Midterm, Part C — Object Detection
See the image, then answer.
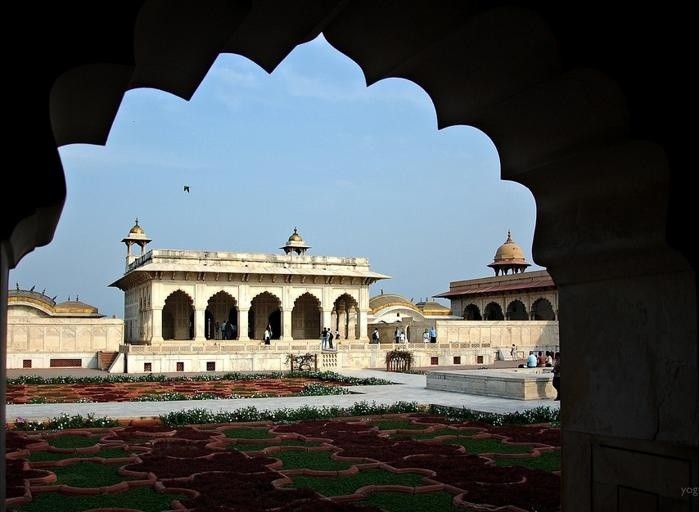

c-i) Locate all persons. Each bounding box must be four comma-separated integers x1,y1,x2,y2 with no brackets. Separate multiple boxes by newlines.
327,328,333,349
537,351,546,367
215,320,237,340
527,351,537,368
335,329,340,339
545,351,553,367
400,332,405,342
372,328,380,343
429,326,436,343
321,327,328,350
552,352,560,401
422,327,429,343
510,344,517,362
395,326,401,343
264,323,273,345
